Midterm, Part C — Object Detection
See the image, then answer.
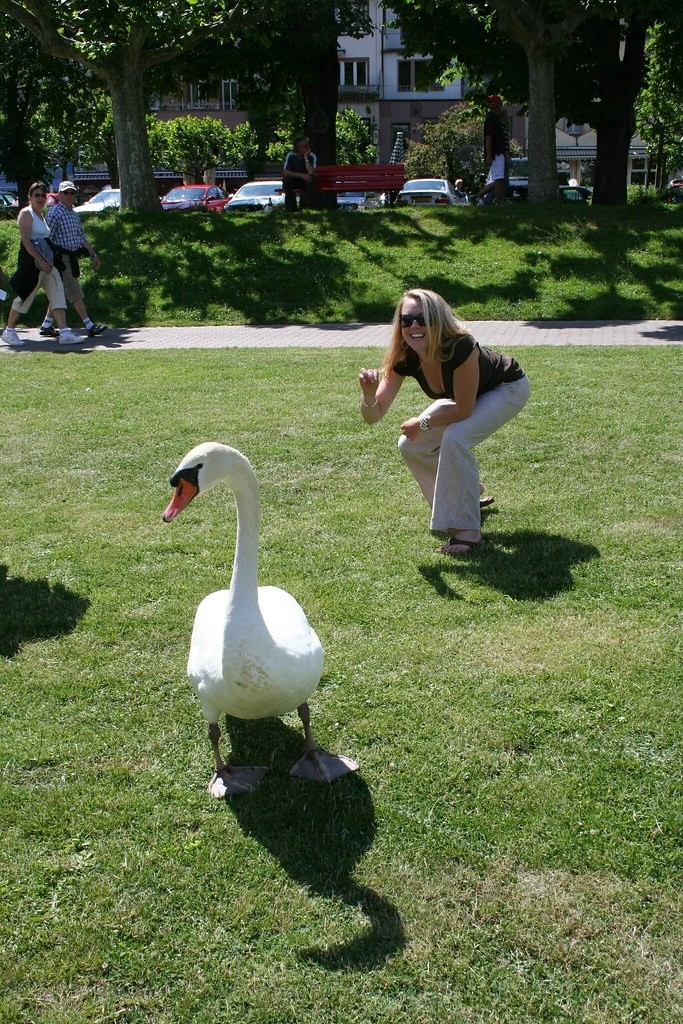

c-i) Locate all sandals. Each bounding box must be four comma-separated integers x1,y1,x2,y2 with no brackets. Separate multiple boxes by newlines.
39,325,60,336
86,324,107,337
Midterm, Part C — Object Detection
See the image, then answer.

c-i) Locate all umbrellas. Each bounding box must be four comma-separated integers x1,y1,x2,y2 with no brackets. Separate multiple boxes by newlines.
389,132,404,164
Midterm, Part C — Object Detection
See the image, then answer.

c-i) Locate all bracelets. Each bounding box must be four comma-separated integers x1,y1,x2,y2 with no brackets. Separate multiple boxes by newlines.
305,160,309,162
91,253,97,260
419,417,432,432
361,394,378,408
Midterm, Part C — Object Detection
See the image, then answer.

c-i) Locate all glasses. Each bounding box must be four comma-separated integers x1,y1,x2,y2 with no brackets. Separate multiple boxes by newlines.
399,312,436,329
64,191,76,196
32,193,46,198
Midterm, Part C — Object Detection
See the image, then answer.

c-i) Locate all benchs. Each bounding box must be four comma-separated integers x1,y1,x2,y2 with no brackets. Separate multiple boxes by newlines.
274,164,405,209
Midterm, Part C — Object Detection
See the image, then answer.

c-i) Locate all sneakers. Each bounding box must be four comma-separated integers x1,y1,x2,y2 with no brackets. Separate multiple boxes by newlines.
468,194,480,206
2,330,26,346
59,333,85,345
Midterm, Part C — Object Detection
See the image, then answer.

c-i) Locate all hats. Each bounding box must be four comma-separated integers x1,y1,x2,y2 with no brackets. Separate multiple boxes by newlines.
489,96,502,105
58,181,78,192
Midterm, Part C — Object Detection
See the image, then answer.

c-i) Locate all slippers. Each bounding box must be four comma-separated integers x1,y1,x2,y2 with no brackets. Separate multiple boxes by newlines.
478,495,494,511
435,536,483,556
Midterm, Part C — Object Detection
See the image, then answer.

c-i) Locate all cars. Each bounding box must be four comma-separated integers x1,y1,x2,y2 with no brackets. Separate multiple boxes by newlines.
161,185,232,213
379,190,398,209
73,189,121,212
11,194,60,207
481,180,593,204
663,179,683,203
337,190,382,210
395,179,466,205
0,191,20,211
223,180,300,212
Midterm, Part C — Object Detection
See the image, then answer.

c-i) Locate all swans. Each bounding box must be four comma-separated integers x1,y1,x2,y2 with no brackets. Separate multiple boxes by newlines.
162,441,362,798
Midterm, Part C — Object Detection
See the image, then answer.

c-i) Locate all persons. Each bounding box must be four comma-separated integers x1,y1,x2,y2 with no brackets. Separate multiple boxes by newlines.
39,181,108,338
470,95,512,206
359,289,530,553
2,181,84,345
568,174,579,187
283,136,316,213
454,179,468,203
